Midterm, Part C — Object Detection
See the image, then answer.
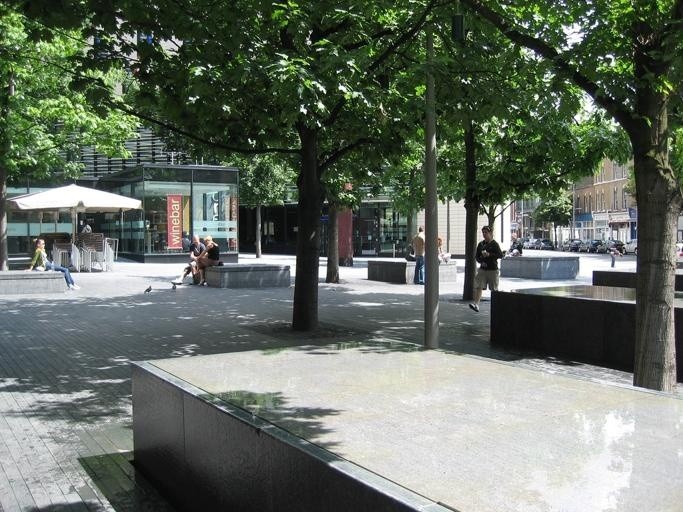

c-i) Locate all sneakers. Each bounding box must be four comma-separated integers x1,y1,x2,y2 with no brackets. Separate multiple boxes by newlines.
171,278,183,285
469,303,480,312
67,283,80,290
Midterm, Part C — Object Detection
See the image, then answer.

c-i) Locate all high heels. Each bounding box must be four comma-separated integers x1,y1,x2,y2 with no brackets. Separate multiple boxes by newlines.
196,280,206,286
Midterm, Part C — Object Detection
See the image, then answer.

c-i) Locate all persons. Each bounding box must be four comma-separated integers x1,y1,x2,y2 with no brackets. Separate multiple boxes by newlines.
172,235,205,285
609,242,623,267
196,235,219,286
80,220,92,233
509,233,522,256
25,239,80,292
469,226,503,312
412,227,425,285
437,238,449,264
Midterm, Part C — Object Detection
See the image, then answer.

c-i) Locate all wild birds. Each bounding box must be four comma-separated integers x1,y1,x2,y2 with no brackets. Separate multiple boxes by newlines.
143,285,152,295
172,283,176,290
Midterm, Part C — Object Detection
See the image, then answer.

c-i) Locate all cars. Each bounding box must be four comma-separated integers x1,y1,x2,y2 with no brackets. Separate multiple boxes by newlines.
675,242,682,263
520,237,637,256
